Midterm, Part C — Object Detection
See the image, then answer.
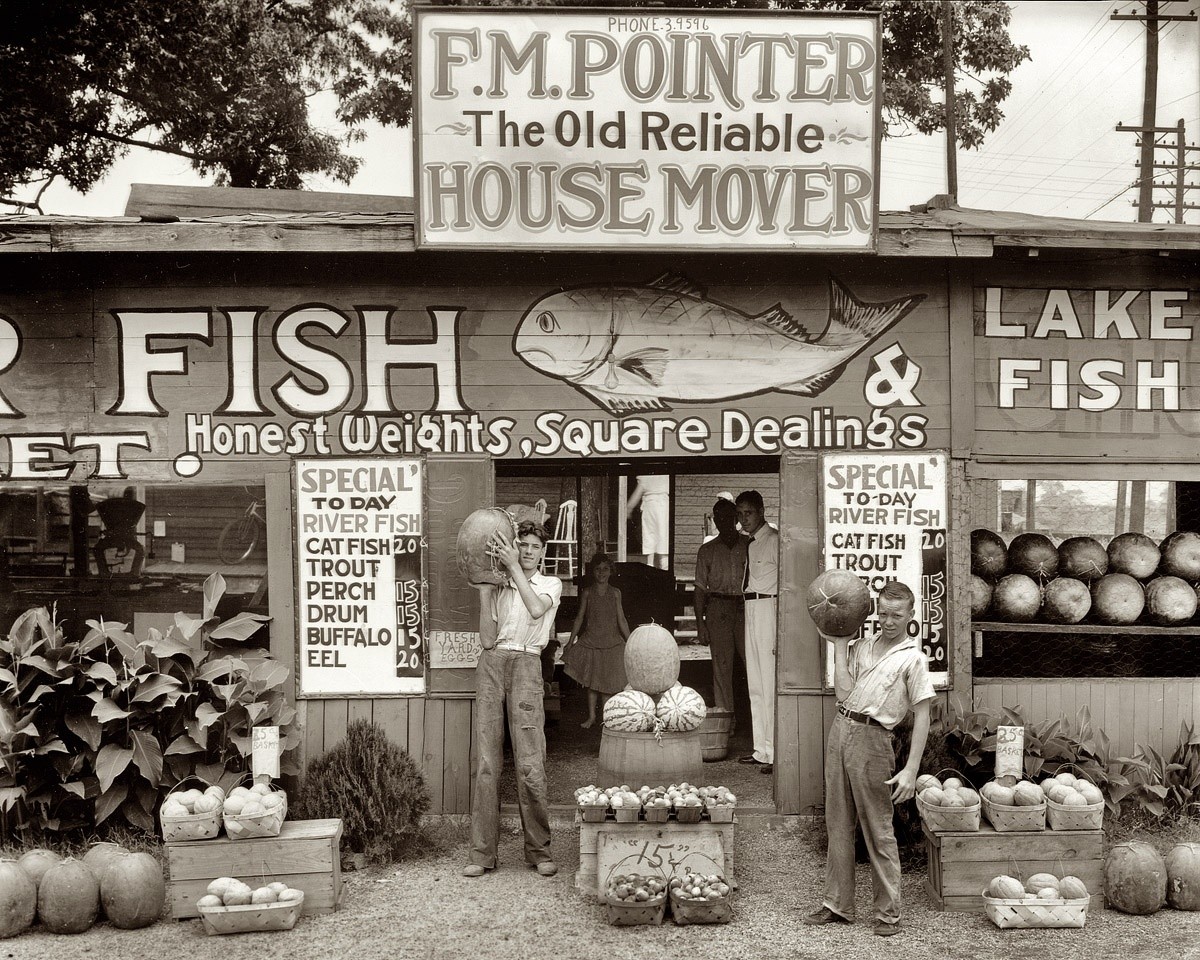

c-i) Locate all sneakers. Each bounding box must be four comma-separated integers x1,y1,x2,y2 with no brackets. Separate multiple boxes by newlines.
804,906,843,925
875,919,901,936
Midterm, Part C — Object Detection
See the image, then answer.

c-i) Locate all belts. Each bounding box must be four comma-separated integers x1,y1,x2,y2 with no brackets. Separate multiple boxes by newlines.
838,706,881,726
711,592,742,601
495,642,542,656
745,593,777,600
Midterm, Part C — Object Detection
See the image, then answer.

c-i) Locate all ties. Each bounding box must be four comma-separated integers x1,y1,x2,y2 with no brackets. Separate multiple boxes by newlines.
743,536,755,593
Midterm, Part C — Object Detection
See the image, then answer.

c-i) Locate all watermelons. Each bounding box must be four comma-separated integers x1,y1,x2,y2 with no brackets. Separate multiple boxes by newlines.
807,569,871,636
969,528,1200,626
603,617,707,732
456,507,517,584
1104,840,1200,914
0,842,166,939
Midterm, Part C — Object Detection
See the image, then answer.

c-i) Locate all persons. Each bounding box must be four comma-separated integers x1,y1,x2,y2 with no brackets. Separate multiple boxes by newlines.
626,475,670,570
93,486,146,579
735,490,781,774
558,551,633,729
692,498,753,737
803,582,938,934
462,521,562,878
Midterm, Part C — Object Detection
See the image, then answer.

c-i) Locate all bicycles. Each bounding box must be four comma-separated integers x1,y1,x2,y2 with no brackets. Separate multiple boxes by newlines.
218,484,266,565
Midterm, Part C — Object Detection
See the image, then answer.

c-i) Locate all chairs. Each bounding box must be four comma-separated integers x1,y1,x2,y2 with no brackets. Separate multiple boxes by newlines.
532,497,580,578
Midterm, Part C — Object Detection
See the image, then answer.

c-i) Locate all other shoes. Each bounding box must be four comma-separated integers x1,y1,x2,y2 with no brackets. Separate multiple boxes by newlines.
536,861,558,876
738,756,764,764
761,765,773,774
464,865,484,877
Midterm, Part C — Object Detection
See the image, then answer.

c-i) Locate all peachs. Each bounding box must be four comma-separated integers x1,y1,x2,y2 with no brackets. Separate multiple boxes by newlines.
608,874,666,903
670,872,728,900
574,784,737,807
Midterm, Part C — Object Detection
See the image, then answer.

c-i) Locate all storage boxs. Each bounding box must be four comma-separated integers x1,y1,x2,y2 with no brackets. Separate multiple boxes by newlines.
162,817,349,921
922,820,1113,918
573,806,740,896
